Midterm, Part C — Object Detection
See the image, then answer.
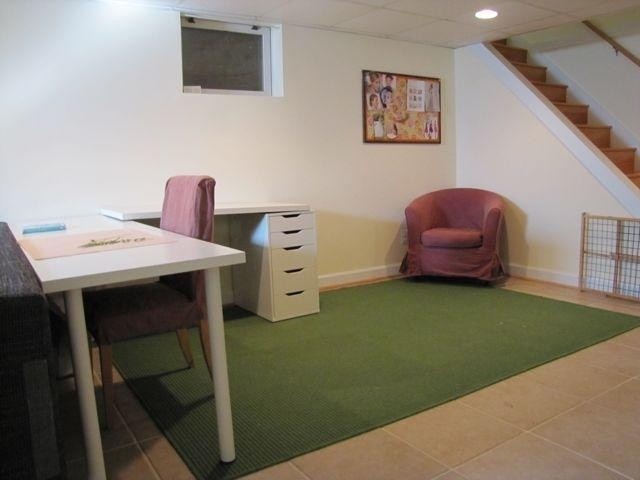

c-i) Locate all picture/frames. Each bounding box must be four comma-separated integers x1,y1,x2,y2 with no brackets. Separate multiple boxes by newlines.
362,68,442,145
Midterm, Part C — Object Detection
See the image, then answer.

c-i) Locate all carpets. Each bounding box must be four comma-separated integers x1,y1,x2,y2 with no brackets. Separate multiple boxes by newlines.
108,277,639,480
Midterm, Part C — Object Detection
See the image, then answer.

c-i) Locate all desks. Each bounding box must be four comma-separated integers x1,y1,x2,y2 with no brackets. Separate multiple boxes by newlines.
98,201,319,322
6,215,246,480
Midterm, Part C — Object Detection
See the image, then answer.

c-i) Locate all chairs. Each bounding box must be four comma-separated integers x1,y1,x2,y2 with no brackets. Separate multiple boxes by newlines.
398,188,506,289
64,176,216,432
